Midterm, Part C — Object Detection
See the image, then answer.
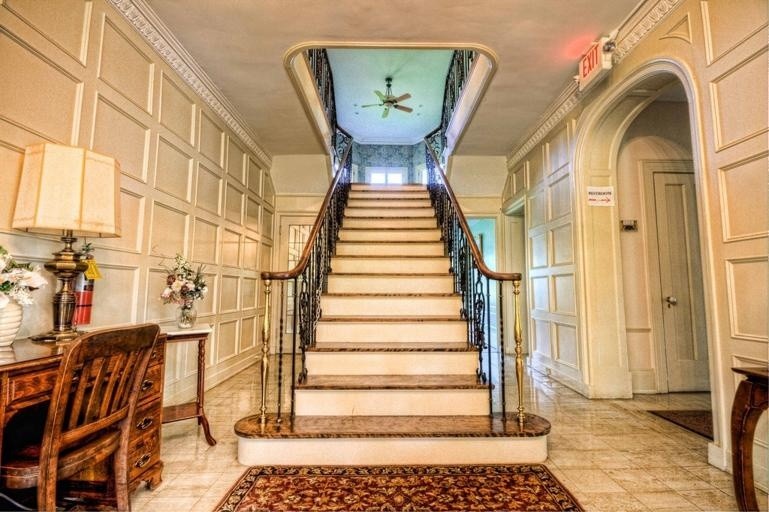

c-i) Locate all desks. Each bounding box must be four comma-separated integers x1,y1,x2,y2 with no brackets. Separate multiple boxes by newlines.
151,321,218,446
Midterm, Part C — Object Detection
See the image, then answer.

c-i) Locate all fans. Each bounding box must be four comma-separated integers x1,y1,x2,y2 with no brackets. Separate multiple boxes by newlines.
361,77,414,119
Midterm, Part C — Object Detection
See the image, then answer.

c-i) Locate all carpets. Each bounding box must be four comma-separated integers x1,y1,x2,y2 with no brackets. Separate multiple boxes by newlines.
646,409,713,440
210,465,588,512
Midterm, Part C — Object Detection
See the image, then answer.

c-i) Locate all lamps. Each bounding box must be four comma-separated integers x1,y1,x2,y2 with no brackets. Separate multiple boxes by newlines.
12,142,123,344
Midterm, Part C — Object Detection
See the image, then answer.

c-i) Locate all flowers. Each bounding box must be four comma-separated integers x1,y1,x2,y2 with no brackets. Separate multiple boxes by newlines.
0,246,49,309
157,251,209,325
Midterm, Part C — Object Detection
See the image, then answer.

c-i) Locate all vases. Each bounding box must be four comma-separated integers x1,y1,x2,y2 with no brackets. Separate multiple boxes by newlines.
175,304,197,329
0,298,23,346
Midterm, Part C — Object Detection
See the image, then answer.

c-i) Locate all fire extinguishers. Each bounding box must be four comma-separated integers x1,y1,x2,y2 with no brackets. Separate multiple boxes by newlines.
72,241,95,327
